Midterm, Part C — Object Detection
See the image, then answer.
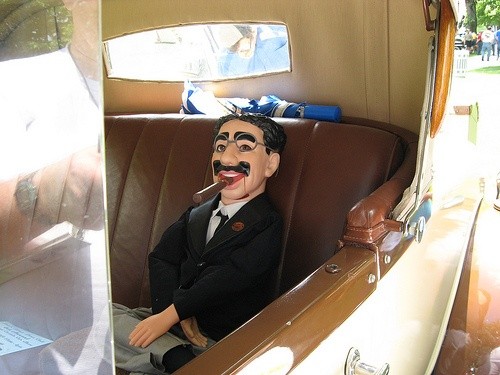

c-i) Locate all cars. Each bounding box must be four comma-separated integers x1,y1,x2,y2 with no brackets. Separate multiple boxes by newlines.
1,1,487,375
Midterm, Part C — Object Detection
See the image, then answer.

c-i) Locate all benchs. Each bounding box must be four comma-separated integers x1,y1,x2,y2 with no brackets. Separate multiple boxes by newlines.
0,114,419,375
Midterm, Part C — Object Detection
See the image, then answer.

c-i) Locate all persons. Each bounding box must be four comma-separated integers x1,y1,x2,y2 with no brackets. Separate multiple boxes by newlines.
455,27,500,61
128,113,286,354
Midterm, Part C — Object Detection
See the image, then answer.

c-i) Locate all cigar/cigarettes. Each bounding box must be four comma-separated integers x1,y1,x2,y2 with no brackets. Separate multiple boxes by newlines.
191,177,234,203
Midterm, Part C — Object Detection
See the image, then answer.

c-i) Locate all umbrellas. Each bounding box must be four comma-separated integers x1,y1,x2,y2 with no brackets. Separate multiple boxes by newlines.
179,81,343,124
